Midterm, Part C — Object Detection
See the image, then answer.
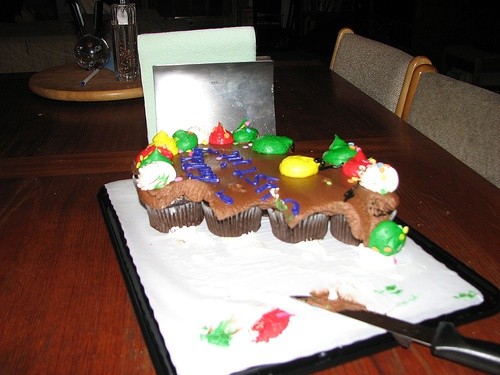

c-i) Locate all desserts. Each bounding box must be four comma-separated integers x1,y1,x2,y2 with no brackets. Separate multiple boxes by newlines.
130,118,409,256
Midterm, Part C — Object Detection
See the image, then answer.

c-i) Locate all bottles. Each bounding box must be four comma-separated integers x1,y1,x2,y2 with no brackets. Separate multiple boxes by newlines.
110,0,140,81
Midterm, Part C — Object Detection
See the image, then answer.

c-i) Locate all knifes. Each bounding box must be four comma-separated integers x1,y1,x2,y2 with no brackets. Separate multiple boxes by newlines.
291,296,500,375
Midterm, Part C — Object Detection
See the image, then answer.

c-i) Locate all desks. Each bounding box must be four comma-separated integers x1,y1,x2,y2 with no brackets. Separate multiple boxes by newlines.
0,58,500,374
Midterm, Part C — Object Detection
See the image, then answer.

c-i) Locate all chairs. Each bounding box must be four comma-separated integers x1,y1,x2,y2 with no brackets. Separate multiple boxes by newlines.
327,27,500,190
